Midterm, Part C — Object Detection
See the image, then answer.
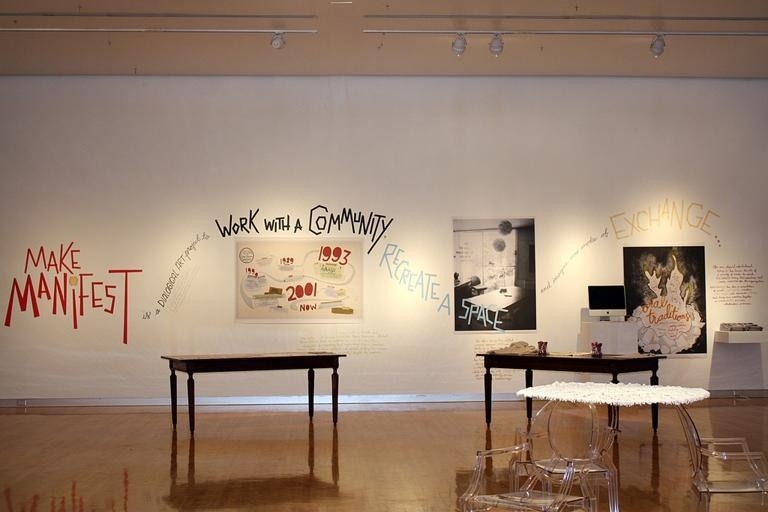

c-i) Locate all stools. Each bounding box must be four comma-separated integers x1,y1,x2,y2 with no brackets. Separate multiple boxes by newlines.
470,286,487,296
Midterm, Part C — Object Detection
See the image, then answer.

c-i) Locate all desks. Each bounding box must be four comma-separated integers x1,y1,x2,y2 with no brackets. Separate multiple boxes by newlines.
464,285,531,322
475,350,667,432
162,352,347,433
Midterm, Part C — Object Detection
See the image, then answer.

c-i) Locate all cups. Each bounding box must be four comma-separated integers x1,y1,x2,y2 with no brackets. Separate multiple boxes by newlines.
537,341,548,354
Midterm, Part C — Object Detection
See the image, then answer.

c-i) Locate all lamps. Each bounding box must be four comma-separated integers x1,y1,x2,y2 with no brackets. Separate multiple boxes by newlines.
488,33,504,55
452,35,466,53
271,33,284,49
650,37,667,56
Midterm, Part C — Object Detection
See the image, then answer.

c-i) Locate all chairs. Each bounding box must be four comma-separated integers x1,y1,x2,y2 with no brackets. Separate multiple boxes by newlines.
457,400,600,512
675,403,768,512
516,403,620,512
